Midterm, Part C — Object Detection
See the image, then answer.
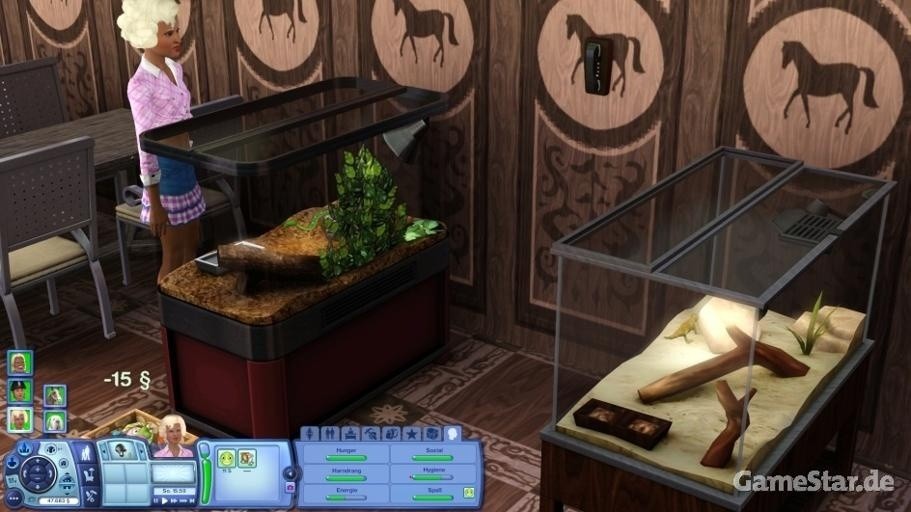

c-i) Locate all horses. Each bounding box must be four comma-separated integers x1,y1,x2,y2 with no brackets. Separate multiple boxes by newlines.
259,0,307,42
566,16,645,97
782,42,879,134
393,0,459,67
